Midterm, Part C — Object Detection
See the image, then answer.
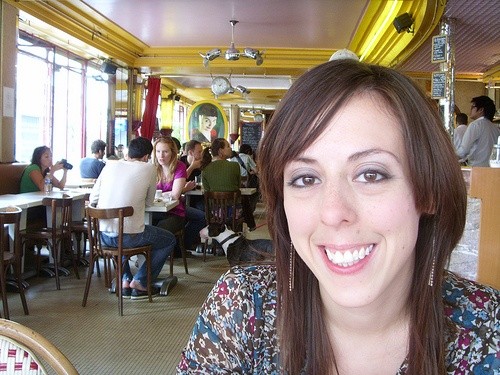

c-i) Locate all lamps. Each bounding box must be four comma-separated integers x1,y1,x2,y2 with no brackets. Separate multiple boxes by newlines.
200,19,264,66
228,86,246,93
99,58,119,74
393,14,413,33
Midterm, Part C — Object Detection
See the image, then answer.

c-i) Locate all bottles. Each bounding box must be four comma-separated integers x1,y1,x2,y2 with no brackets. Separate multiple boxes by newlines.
43,172,53,196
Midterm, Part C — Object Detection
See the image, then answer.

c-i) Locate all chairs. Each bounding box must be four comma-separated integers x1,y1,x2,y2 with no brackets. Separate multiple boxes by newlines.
1,318,80,375
202,191,244,260
0,193,152,321
170,193,189,277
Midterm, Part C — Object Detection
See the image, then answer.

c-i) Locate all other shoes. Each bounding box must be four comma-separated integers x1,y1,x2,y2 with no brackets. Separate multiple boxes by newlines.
121,288,131,298
173,251,191,257
131,288,157,299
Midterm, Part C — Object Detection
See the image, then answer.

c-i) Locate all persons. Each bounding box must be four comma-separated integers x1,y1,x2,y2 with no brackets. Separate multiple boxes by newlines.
79,139,106,179
89,137,174,300
175,59,500,375
20,145,67,229
151,138,188,234
191,104,218,143
454,95,500,167
171,137,259,253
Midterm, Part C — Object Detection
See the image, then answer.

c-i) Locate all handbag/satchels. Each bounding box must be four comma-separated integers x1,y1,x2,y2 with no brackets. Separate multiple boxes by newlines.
246,174,259,199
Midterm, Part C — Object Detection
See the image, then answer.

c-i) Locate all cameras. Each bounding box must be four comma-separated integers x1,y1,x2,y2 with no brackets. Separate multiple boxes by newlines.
59,159,72,169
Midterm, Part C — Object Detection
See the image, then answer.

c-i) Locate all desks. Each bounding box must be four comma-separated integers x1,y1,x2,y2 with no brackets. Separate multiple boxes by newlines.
109,198,180,296
183,188,257,253
0,182,95,292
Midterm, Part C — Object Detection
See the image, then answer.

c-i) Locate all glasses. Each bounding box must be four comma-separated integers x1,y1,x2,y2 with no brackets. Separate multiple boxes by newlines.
471,106,476,110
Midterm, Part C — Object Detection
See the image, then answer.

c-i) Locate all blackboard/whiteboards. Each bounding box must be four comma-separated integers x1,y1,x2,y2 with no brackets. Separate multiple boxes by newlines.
431,34,448,63
239,121,263,152
431,71,446,99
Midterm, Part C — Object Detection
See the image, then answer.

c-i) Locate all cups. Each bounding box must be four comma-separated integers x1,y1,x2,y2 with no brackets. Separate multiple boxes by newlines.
155,190,162,199
195,176,201,189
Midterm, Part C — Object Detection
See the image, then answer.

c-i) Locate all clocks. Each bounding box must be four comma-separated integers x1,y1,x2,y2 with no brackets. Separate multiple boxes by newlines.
211,76,231,96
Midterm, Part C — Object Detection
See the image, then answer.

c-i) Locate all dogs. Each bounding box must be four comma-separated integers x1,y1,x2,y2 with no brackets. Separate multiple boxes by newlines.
199,222,277,266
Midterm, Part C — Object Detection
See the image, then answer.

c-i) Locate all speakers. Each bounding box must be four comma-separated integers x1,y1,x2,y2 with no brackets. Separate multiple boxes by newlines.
102,62,117,74
393,12,413,34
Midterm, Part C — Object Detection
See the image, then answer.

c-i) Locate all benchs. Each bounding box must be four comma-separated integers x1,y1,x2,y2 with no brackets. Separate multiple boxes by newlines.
0,162,46,251
